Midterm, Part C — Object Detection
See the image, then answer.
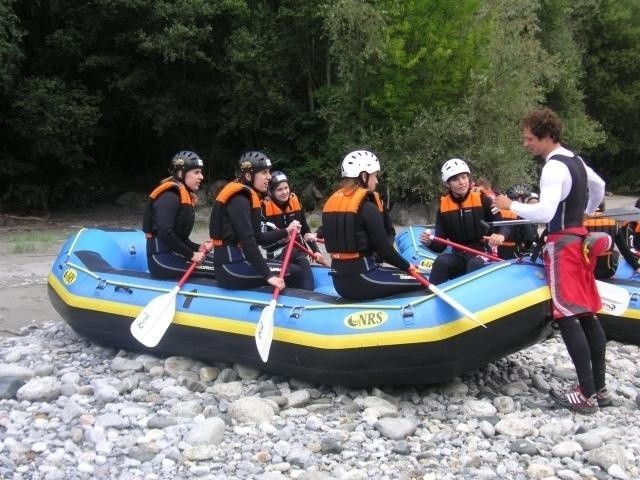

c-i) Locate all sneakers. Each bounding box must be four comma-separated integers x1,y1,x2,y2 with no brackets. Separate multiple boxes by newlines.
549,384,612,414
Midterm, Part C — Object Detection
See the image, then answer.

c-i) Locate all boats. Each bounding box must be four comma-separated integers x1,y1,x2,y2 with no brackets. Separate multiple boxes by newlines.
47,226,554,389
394,215,640,348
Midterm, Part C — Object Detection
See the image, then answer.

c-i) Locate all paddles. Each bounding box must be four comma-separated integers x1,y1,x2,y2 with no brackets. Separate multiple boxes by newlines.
431,234,630,316
254,228,297,363
129,245,207,349
411,267,487,329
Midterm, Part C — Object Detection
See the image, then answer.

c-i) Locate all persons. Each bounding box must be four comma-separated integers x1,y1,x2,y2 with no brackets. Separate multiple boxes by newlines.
470,178,640,279
420,158,510,286
210,150,314,291
142,150,215,280
304,190,396,263
258,171,328,290
494,108,612,413
322,150,428,301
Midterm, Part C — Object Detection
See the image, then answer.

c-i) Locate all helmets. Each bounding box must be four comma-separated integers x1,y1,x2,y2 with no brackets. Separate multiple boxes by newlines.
506,184,530,200
236,151,288,189
341,150,380,178
169,151,204,181
440,159,470,182
